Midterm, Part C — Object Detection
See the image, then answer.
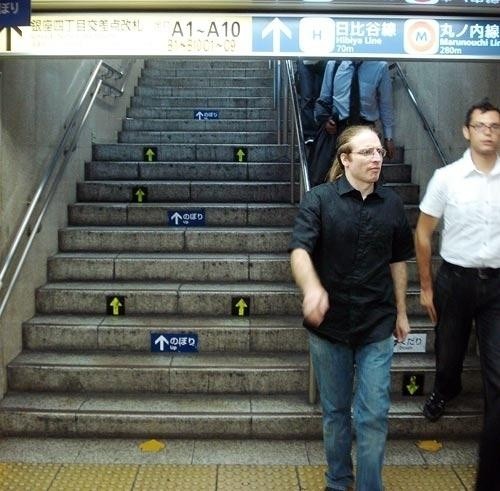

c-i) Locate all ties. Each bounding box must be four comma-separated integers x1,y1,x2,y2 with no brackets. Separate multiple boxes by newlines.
349,60,364,125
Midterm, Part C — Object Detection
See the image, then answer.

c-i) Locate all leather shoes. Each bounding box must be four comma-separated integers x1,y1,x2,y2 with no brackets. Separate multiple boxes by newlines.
423,388,448,423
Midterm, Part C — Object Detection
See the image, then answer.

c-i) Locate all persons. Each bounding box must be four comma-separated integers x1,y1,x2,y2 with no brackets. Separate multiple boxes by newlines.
287,126,417,491
314,59,395,182
413,100,500,491
298,58,326,144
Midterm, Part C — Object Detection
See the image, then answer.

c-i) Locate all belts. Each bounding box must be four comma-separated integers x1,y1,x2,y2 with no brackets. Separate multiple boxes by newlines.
446,266,500,280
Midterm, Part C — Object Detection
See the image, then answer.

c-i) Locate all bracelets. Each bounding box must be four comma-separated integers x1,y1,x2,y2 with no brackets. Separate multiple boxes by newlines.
384,137,394,142
